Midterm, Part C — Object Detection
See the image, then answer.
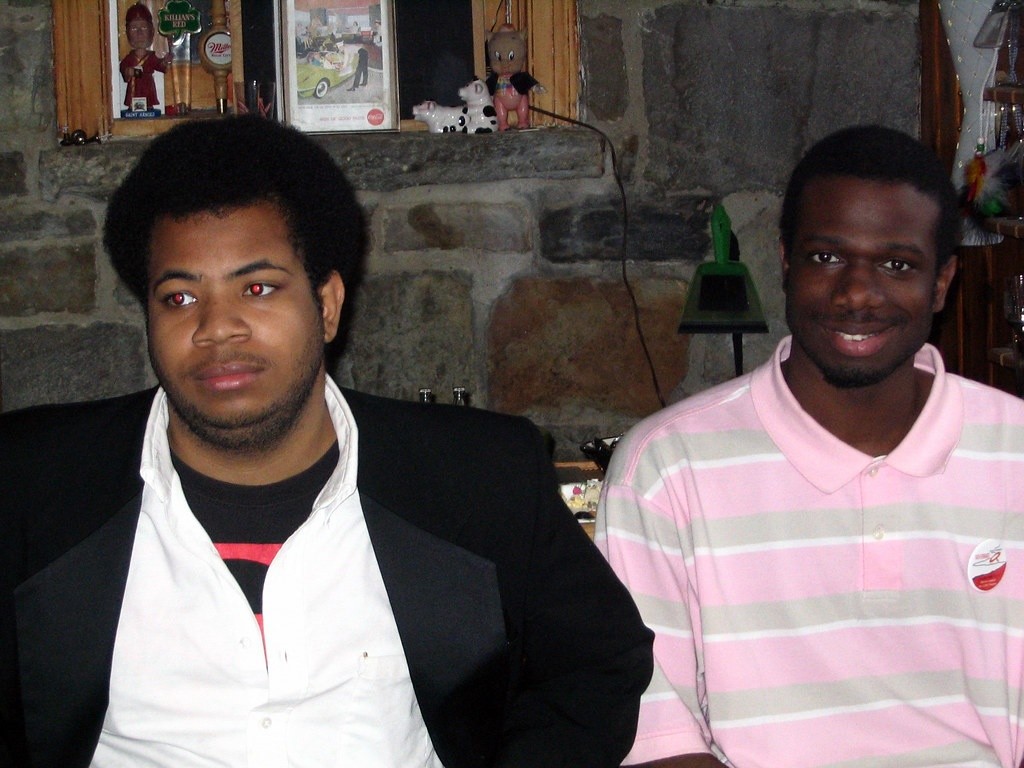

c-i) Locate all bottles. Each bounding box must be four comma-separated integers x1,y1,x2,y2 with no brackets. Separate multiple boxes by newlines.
451,386,466,406
419,388,431,404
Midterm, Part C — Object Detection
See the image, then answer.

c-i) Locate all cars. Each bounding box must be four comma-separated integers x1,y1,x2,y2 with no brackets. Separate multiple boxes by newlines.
296,44,360,99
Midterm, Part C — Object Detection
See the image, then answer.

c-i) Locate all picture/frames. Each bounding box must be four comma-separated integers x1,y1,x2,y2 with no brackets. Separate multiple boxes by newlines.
280,0,402,135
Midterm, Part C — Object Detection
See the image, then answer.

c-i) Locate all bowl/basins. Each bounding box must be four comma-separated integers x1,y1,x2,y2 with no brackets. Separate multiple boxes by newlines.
580,436,623,475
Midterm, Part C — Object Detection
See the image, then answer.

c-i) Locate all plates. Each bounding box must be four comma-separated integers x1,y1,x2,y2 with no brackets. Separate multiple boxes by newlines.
558,481,596,523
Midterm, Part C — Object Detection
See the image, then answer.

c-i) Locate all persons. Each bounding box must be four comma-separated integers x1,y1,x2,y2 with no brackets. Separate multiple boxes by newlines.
594,124,1024,768
120,3,173,111
0,113,654,768
